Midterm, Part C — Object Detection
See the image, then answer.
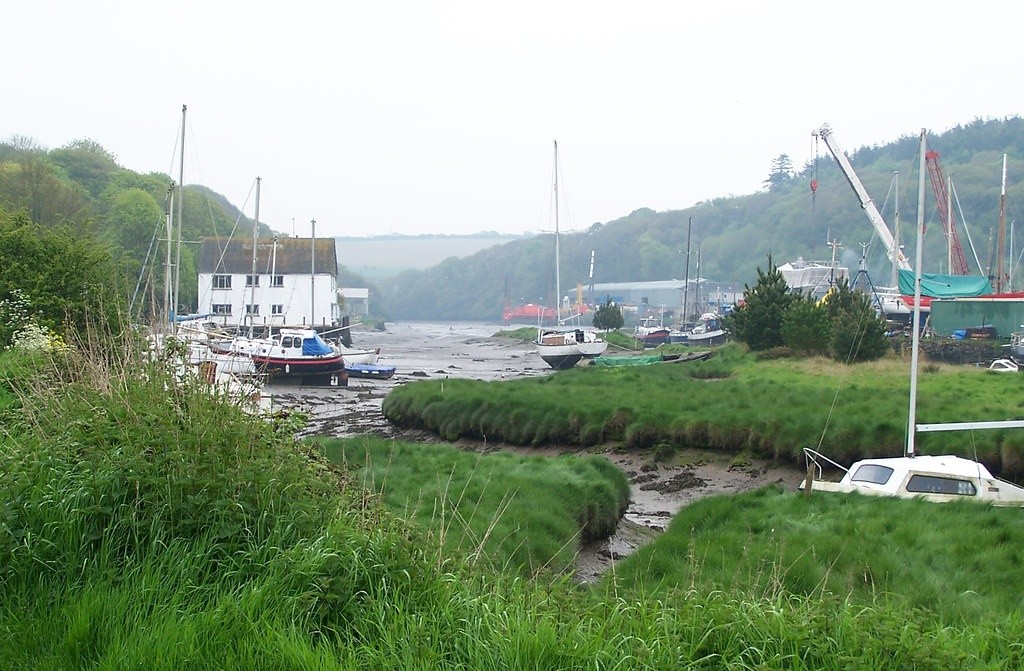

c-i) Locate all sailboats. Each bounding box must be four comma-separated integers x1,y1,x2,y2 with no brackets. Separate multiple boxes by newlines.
532,141,608,371
797,128,1024,511
119,104,397,413
667,217,735,348
853,169,912,329
897,172,995,331
930,154,1024,340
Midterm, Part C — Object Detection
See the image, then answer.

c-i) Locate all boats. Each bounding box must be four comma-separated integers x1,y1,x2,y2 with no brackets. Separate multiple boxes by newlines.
219,328,349,387
773,227,851,304
633,306,670,349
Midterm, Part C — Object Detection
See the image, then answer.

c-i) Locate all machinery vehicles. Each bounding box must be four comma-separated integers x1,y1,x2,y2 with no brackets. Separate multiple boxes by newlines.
812,123,913,272
